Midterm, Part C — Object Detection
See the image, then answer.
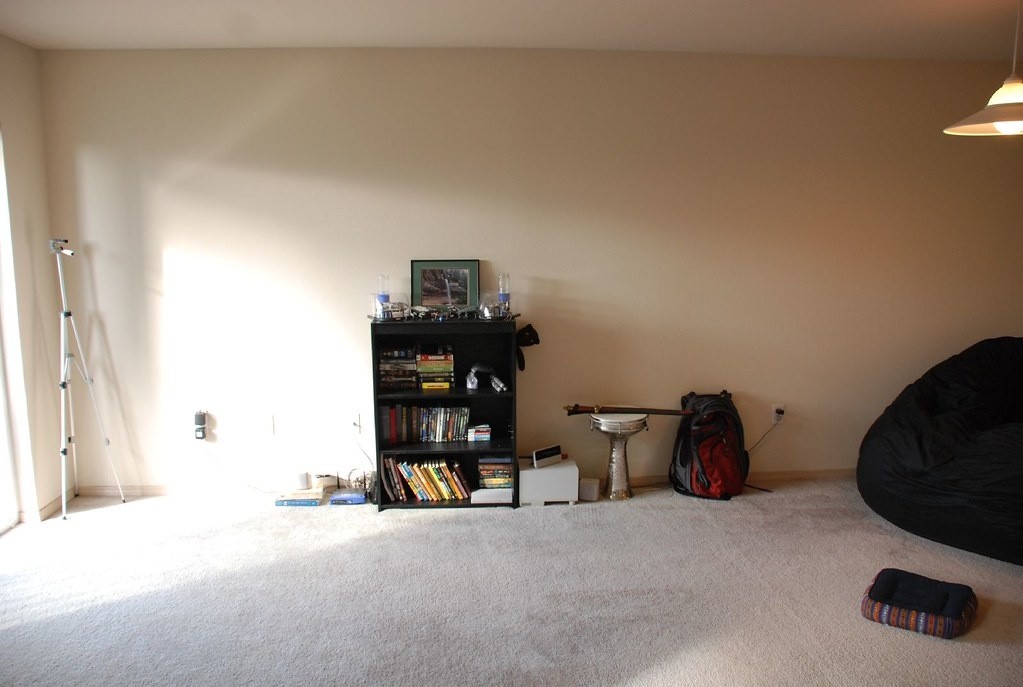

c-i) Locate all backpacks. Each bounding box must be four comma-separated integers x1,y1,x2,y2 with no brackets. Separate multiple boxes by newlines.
670,391,750,501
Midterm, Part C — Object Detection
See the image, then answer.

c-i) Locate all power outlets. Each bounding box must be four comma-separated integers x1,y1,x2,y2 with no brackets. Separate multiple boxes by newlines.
771,403,785,426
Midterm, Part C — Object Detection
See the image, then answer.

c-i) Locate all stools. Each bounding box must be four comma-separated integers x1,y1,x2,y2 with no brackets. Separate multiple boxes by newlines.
518,458,579,508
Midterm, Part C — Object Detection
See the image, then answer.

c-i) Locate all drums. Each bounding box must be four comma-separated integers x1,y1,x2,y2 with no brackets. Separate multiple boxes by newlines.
589,413,649,500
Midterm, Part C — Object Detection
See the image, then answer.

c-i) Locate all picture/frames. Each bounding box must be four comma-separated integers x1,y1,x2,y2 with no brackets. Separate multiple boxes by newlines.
410,259,479,312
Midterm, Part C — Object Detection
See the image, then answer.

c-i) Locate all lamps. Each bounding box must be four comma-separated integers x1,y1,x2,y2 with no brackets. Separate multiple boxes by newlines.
943,0,1023,136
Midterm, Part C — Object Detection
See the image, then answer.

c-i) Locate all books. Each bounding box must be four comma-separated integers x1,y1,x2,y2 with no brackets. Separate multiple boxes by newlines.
378,340,455,390
377,401,491,444
275,489,325,506
379,452,514,503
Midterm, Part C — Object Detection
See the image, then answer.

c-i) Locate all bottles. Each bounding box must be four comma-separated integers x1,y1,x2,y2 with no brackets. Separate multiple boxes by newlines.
498,272,510,307
377,273,389,304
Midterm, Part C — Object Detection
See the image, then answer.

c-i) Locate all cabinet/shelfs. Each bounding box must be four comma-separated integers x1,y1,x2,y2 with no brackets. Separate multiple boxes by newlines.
370,318,520,513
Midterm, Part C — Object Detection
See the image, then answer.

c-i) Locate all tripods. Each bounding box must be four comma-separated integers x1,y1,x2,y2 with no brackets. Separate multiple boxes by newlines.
56,252,126,519
